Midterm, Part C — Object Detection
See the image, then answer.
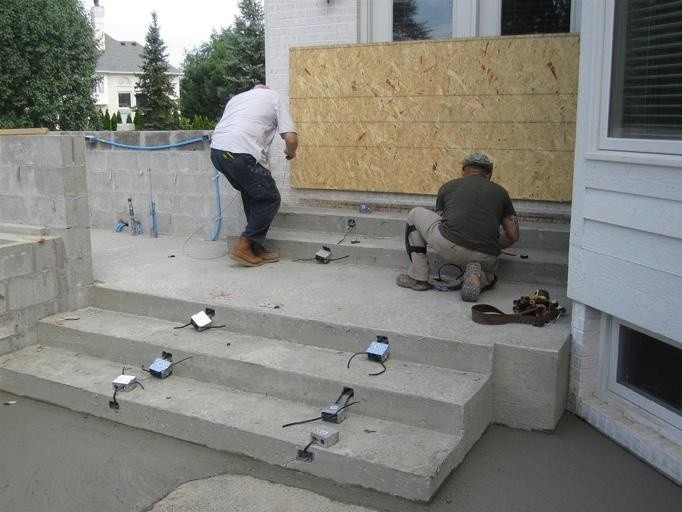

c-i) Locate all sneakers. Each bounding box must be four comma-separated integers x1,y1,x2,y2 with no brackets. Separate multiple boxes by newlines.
396,274,430,291
461,261,482,302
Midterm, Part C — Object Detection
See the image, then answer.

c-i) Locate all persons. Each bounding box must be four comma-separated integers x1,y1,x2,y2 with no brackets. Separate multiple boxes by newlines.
394,151,521,302
209,82,299,267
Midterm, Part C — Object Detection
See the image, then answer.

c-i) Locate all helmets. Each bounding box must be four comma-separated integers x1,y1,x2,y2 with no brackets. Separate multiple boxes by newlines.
462,152,493,179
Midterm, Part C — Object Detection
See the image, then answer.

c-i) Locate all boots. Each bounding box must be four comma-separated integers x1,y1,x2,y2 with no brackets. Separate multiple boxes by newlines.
228,235,279,267
